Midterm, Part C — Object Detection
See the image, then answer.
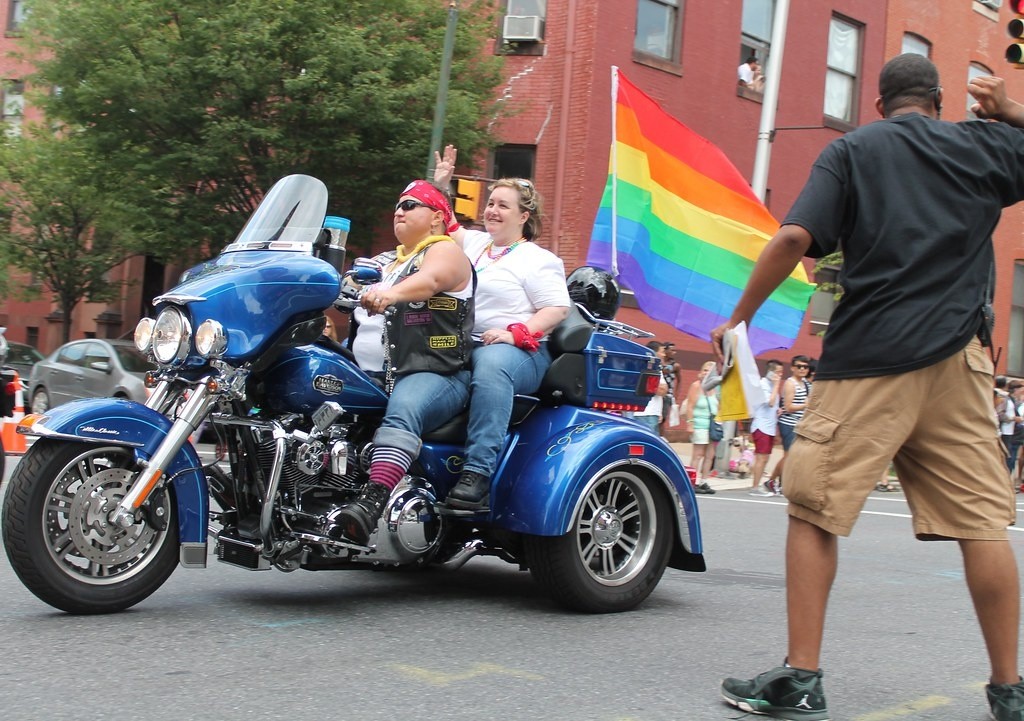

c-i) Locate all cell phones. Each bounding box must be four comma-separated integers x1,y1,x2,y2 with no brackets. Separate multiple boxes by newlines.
766,371,775,380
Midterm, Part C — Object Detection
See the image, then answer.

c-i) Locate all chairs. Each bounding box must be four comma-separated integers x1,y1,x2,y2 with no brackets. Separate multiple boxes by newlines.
511,298,593,426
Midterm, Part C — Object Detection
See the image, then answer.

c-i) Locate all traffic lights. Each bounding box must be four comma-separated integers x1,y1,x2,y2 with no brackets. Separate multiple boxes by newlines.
1004,0,1024,70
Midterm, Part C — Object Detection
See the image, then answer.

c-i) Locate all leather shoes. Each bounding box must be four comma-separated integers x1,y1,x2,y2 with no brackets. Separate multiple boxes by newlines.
445,470,490,510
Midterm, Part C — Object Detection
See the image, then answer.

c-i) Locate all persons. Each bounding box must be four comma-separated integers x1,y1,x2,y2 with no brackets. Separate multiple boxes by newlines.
873,466,900,492
804,358,817,383
994,375,1024,494
435,144,570,509
763,355,817,497
337,181,478,546
747,360,782,497
323,315,337,342
685,356,726,496
633,341,682,444
710,52,1024,721
737,56,765,93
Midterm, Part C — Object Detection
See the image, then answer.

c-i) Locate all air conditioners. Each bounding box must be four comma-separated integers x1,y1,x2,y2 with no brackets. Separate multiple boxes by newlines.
503,16,543,41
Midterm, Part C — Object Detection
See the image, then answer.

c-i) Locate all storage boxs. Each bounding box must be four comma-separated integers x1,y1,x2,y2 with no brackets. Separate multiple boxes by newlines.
582,332,660,412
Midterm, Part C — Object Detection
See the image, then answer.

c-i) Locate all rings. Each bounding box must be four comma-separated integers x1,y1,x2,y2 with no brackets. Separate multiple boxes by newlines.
495,333,499,337
375,298,381,302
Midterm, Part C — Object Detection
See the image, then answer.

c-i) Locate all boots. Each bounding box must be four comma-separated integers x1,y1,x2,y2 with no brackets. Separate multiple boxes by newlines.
334,481,390,543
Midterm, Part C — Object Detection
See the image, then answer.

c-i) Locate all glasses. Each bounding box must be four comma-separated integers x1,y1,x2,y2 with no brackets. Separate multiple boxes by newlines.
517,180,532,196
793,364,810,369
396,199,438,210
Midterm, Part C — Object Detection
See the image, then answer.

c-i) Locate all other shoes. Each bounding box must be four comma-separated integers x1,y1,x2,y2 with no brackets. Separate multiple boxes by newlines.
748,488,768,496
696,483,715,494
764,480,778,495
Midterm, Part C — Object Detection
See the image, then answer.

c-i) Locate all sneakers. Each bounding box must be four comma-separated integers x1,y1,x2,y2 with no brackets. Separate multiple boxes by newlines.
986,676,1024,721
721,656,831,721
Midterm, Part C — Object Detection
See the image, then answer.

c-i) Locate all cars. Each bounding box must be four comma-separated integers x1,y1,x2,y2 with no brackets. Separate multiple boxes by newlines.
2,341,45,409
27,338,228,443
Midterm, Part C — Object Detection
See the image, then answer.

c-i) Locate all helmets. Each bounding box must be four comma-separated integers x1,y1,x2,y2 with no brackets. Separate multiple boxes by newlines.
566,266,621,331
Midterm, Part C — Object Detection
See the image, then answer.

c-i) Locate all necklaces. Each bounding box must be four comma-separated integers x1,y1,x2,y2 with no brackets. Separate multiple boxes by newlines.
474,236,528,274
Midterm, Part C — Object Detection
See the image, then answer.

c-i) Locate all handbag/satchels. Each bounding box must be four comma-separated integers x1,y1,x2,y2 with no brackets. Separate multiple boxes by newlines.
1011,409,1024,446
709,415,724,442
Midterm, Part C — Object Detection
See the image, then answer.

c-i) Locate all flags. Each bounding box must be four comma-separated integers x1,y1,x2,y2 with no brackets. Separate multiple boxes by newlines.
584,67,818,357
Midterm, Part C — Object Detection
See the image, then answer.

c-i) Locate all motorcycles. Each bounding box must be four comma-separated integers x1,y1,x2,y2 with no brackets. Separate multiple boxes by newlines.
0,175,708,614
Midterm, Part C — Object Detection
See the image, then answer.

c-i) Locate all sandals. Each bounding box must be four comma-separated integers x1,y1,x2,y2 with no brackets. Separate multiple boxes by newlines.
877,483,900,492
